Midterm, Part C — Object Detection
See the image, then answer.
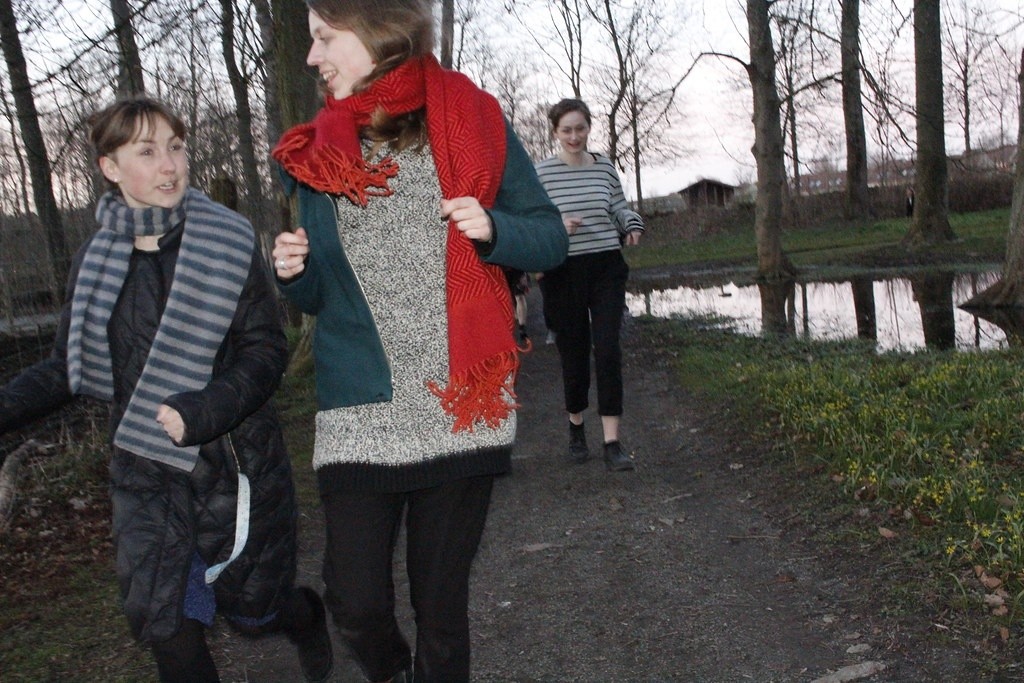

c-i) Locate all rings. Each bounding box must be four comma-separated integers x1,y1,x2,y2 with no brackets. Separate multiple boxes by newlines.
276,256,287,270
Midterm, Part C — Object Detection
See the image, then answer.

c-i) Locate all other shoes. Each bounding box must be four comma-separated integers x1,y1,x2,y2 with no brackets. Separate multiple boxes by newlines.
289,587,334,683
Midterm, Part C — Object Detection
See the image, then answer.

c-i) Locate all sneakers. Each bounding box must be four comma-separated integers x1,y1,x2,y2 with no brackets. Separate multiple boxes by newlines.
603,439,635,471
568,419,589,463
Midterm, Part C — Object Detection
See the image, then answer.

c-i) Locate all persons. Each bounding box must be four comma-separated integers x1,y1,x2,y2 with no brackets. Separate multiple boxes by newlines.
509,270,529,341
273,0,570,682
532,97,646,476
1,100,332,683
531,272,559,346
